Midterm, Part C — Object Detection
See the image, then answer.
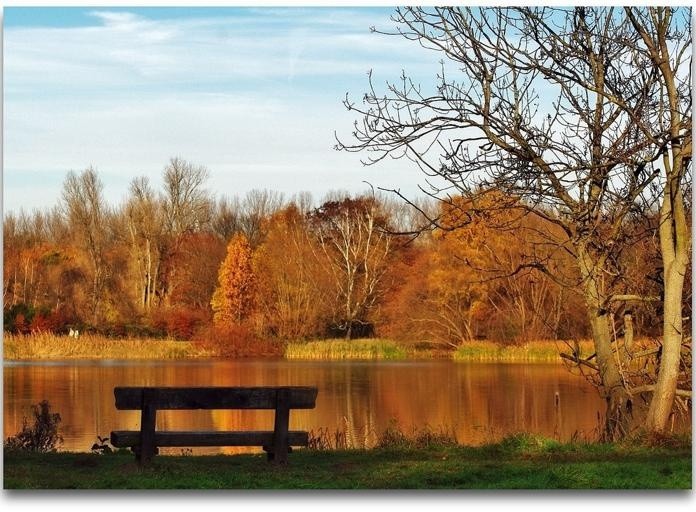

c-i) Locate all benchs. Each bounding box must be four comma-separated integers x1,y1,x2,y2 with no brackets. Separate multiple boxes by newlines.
111,386,319,468
